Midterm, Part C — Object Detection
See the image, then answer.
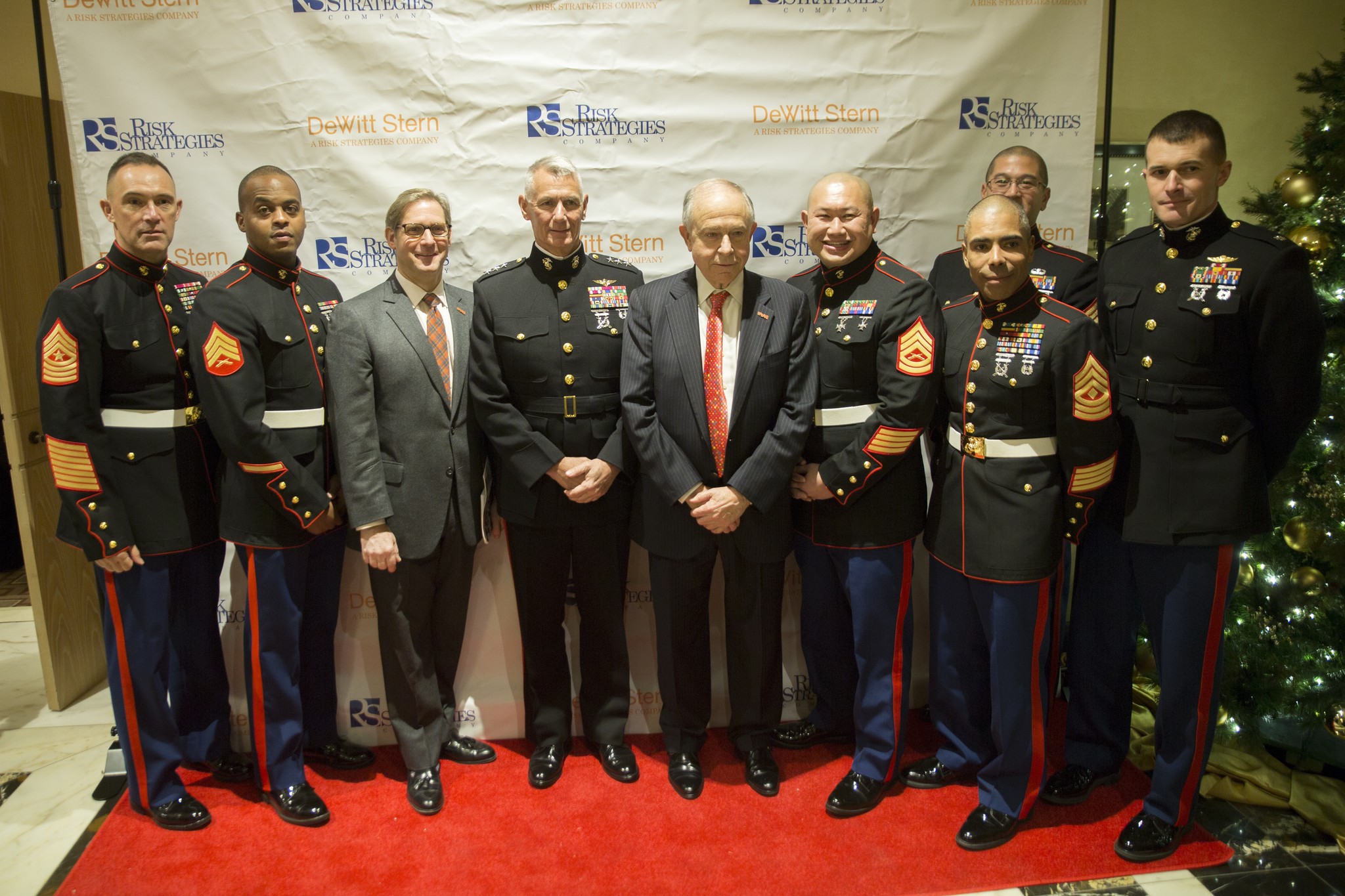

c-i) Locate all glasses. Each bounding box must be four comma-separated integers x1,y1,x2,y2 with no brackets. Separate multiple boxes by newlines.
395,223,452,237
986,177,1047,190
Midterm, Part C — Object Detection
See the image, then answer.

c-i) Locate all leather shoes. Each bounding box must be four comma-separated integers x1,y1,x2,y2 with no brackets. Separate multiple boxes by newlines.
528,741,573,789
304,738,376,771
1040,761,1121,807
131,792,211,831
258,782,330,828
596,743,639,783
896,754,978,788
920,702,931,722
669,753,704,800
825,768,897,817
1115,810,1194,862
736,743,779,796
956,804,1035,850
770,717,856,751
180,746,254,784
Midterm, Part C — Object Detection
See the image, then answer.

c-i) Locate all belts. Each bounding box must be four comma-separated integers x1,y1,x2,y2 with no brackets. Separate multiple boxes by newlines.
947,427,1058,460
813,403,876,427
100,407,201,430
261,408,324,430
520,395,620,419
1115,373,1247,407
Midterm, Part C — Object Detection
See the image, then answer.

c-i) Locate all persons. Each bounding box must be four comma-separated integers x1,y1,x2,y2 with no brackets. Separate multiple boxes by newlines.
1044,110,1314,862
36,153,259,828
189,166,375,823
901,147,1117,850
620,178,819,797
326,189,507,814
782,174,939,817
472,157,647,787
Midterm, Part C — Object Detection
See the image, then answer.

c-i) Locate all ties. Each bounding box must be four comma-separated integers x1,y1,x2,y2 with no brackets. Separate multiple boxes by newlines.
701,291,730,478
423,293,450,405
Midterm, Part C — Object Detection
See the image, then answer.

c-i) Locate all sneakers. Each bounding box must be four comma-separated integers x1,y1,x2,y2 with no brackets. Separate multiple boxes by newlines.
407,763,444,815
441,735,497,764
